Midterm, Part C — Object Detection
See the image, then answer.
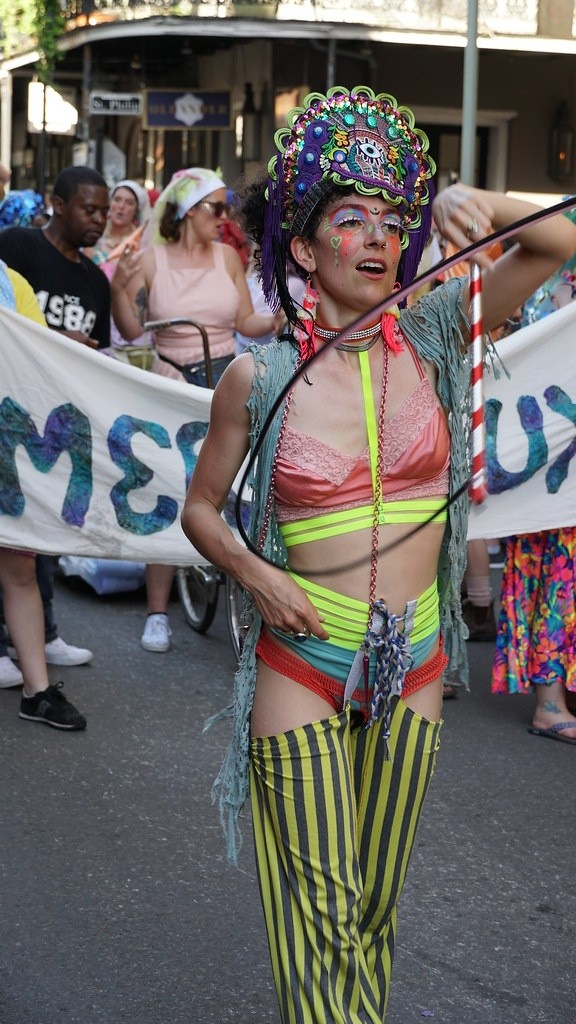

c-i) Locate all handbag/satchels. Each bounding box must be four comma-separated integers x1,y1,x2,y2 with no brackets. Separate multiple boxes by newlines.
158,352,236,390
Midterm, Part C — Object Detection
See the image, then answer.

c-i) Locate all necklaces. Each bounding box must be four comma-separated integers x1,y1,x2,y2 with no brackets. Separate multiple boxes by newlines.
311,317,382,340
321,329,384,353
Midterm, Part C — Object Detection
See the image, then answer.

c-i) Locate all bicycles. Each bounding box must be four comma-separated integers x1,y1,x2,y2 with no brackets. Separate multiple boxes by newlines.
147,319,254,666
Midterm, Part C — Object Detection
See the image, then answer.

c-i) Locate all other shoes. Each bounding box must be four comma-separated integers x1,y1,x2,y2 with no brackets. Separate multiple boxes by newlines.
442,685,458,697
460,598,496,643
488,552,506,568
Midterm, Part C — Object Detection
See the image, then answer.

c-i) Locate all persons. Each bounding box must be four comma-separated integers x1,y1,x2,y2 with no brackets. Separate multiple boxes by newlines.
179,85,576,1022
0,166,576,743
113,167,286,650
0,258,87,731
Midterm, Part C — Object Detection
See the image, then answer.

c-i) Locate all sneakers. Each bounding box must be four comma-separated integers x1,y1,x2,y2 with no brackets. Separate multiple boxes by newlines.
19,681,86,731
0,656,23,689
6,637,93,666
140,612,173,652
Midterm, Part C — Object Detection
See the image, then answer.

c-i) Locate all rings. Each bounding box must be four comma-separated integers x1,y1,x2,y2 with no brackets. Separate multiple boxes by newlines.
465,218,479,240
291,625,309,642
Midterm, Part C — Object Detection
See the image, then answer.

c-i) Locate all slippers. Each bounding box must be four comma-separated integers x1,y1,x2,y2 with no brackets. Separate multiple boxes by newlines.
527,719,576,745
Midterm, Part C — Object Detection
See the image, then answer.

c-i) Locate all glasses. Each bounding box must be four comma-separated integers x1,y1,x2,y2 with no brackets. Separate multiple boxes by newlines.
202,201,231,217
